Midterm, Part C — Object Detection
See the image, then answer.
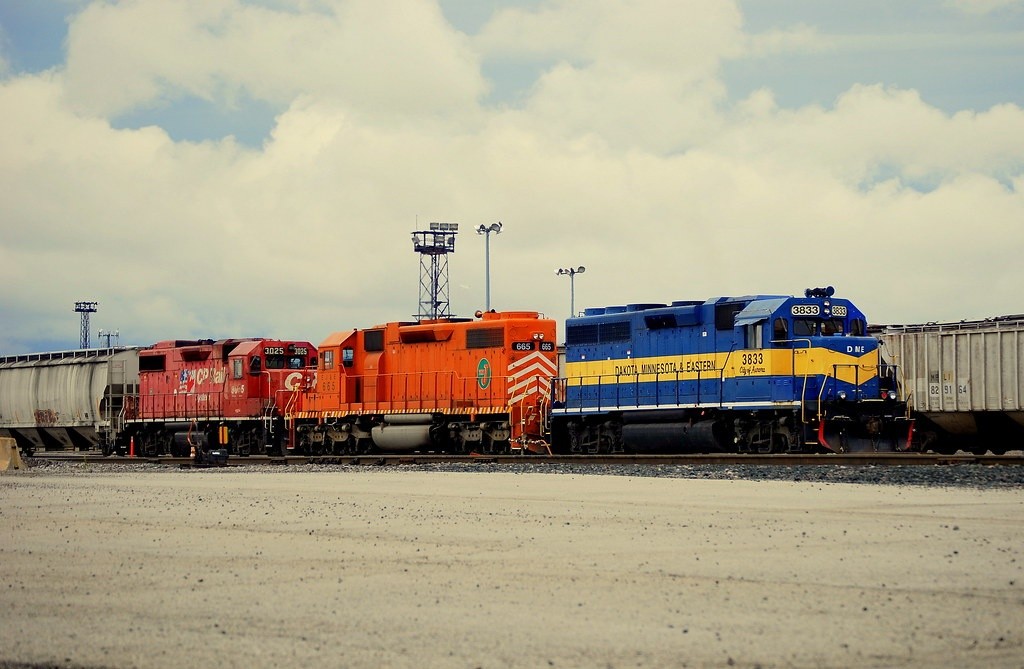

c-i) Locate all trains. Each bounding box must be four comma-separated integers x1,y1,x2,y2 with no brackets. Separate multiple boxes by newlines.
0,284,914,456
863,307,1023,456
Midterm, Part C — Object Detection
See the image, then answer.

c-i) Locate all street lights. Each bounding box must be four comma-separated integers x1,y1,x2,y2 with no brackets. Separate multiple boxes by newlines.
476,220,504,313
553,263,585,319
